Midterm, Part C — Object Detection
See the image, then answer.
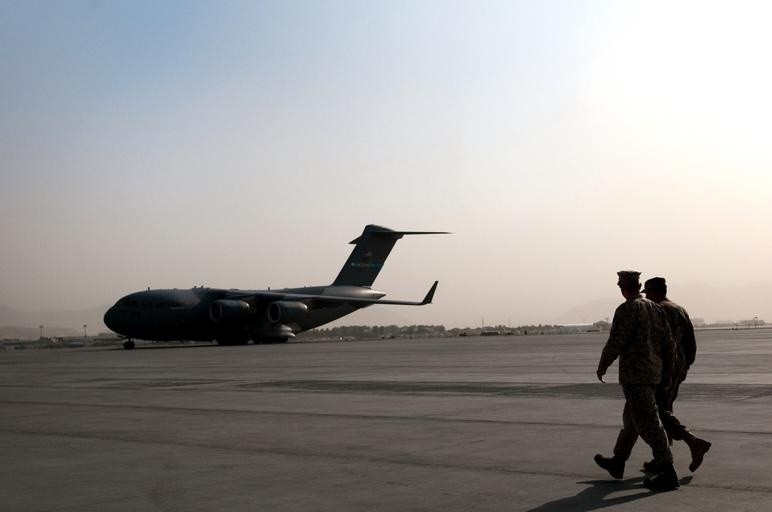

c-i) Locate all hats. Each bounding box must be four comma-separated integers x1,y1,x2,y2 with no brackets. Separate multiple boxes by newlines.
617,272,666,294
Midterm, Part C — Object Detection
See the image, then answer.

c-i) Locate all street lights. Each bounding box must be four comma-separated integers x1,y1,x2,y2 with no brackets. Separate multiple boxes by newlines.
83,324,88,338
40,324,44,336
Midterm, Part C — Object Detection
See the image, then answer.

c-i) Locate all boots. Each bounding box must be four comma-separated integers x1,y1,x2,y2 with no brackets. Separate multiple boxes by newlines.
593,453,626,479
683,432,712,472
643,459,681,488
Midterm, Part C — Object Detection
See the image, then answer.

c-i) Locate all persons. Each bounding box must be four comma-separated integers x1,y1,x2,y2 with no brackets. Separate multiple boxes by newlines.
595,271,712,491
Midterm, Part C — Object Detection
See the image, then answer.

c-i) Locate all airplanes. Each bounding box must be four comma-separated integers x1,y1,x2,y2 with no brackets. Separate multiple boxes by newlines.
96,224,455,350
